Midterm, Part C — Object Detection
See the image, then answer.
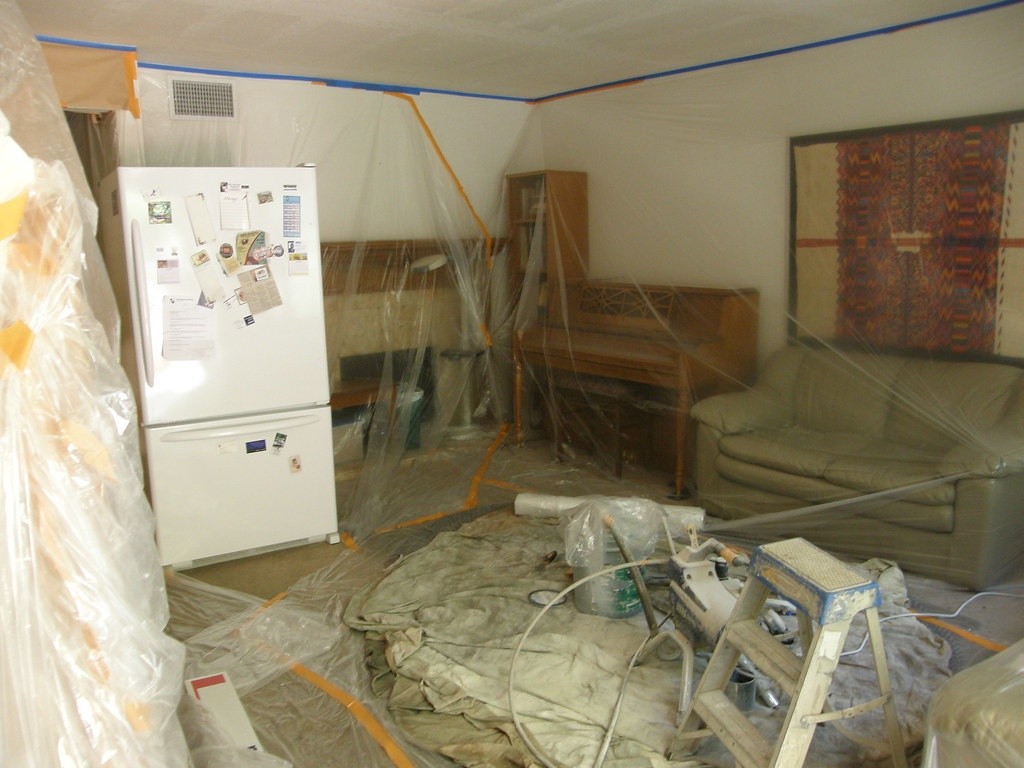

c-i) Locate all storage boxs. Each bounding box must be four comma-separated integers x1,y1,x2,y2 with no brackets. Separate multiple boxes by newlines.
333,417,366,464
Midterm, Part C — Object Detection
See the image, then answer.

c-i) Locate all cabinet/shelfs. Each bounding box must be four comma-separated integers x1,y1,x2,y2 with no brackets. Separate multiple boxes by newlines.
503,171,590,357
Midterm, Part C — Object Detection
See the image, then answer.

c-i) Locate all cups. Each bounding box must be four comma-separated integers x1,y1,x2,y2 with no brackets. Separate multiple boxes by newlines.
724,668,757,711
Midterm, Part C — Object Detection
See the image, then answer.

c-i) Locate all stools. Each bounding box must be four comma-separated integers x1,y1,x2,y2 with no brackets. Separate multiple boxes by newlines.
330,376,399,449
554,374,655,479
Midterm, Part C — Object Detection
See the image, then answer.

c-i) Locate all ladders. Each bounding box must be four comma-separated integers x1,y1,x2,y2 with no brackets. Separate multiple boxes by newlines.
665,538,921,768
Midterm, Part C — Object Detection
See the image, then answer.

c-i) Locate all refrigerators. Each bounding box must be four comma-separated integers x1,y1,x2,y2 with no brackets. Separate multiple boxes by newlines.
97,163,341,570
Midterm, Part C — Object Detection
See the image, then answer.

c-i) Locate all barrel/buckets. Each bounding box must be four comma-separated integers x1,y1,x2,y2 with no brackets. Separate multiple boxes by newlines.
564,499,658,618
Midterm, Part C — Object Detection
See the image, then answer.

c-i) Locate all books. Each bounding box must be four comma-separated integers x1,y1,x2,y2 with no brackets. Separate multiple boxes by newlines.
538,280,547,316
518,224,548,269
518,177,547,221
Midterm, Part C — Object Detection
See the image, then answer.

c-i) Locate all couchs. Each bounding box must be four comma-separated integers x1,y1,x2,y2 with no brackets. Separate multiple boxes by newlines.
690,343,1024,589
